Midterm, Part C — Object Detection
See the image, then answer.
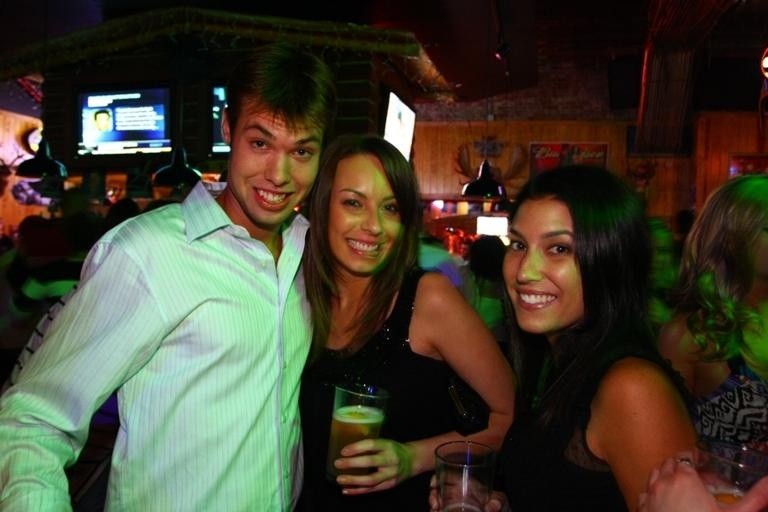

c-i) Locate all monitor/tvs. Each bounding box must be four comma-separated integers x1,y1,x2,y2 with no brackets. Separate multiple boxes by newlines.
372,90,417,169
203,81,238,155
475,213,509,246
75,82,180,153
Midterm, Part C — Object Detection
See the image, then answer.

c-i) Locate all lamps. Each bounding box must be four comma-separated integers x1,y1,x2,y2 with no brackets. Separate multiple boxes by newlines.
459,89,506,199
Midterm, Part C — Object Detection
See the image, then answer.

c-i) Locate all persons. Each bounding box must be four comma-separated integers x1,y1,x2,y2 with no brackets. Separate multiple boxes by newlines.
0,44,767,512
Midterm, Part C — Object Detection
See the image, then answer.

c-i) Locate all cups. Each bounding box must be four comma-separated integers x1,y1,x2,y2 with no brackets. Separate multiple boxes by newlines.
482,202,492,212
689,438,768,506
456,201,468,216
432,438,494,512
324,380,389,488
